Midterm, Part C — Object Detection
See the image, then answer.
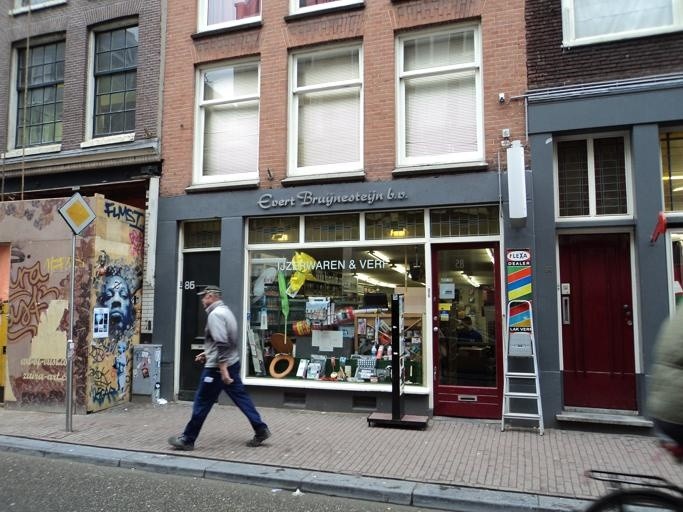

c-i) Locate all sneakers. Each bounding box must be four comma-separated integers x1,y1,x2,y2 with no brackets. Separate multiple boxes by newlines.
168,435,194,451
246,427,272,447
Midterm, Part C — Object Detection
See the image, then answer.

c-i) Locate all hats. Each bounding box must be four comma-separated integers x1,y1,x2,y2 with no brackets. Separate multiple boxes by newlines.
197,285,224,296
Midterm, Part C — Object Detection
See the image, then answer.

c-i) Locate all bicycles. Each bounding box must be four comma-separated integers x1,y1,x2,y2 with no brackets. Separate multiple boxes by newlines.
582,439,683,510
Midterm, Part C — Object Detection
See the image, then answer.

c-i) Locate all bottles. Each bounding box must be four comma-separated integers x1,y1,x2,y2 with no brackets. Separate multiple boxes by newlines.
371,344,392,360
263,269,342,340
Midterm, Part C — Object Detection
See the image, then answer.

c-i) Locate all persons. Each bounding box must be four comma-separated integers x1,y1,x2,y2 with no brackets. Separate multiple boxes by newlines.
642,300,683,449
98,275,135,331
456,316,484,343
167,284,273,453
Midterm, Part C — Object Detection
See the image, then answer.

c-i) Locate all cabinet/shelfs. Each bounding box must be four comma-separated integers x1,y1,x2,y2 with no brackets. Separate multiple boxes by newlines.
247,259,357,356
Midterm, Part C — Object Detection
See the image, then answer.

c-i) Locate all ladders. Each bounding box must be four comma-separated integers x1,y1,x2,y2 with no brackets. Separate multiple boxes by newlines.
501,300,545,435
